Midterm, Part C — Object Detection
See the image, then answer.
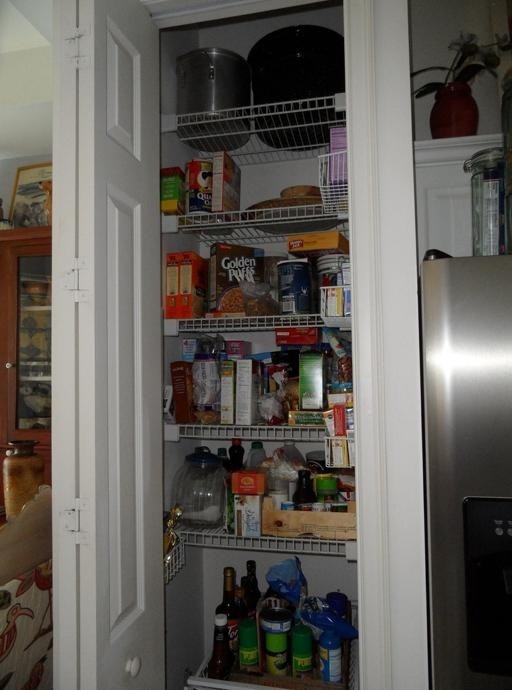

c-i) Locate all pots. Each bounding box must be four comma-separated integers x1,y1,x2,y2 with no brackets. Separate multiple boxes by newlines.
175,47,250,151
247,24,345,151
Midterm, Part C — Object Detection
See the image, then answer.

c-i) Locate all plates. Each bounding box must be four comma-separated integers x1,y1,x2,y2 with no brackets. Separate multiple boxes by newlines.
241,197,348,234
280,185,320,198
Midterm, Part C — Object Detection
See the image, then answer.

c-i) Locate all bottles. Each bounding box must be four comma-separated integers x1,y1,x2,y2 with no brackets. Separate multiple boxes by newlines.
463,147,505,257
316,254,350,318
206,559,343,683
169,443,346,528
276,262,312,320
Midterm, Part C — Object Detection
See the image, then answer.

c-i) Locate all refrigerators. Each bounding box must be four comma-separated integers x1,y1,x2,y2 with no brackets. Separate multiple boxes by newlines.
421,256,511,690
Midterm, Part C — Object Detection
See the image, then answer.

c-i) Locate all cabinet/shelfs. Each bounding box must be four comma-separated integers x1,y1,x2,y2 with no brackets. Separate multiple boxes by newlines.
413,132,506,260
1,227,54,486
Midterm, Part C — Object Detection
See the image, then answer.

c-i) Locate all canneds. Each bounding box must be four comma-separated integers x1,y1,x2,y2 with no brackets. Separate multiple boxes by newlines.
259,608,293,634
277,261,316,325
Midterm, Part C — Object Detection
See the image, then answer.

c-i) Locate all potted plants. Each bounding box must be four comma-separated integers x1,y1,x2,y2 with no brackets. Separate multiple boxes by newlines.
411,26,511,139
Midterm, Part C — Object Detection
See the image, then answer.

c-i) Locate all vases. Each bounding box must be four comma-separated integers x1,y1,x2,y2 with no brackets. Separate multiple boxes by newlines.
3,438,47,523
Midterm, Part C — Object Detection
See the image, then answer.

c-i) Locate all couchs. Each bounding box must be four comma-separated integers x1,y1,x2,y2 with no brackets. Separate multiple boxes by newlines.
0,485,52,689
